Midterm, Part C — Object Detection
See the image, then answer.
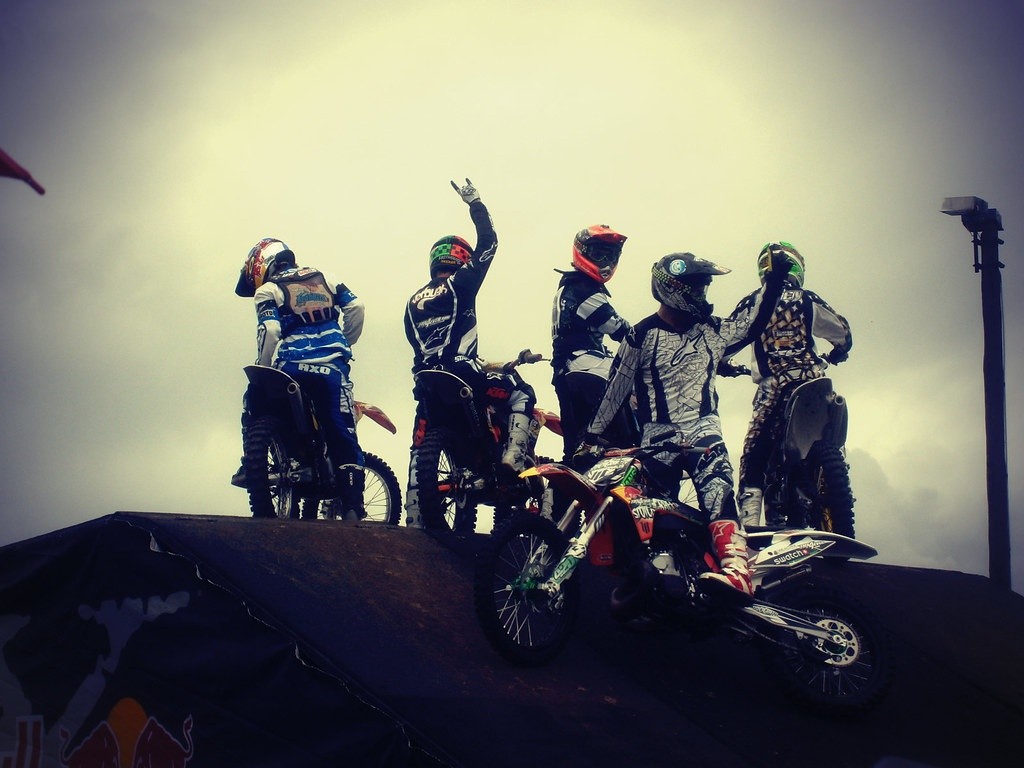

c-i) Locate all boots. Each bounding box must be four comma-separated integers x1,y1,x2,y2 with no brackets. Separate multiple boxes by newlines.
337,464,365,521
404,488,426,529
696,520,753,599
231,469,247,488
498,410,542,494
736,486,763,529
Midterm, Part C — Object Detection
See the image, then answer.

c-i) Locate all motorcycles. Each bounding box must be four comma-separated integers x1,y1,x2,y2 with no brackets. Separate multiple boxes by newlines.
477,433,890,747
238,363,403,527
409,349,564,550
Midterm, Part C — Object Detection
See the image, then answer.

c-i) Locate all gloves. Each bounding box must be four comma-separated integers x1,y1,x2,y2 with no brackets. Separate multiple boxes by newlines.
450,178,481,205
766,245,794,279
571,438,598,466
829,349,849,365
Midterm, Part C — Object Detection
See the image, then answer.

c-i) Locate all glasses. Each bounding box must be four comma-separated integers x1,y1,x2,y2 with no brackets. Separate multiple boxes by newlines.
686,277,711,301
584,244,620,263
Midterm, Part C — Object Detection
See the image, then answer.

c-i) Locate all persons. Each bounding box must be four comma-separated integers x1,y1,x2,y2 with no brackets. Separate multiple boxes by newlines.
718,240,851,528
403,180,537,494
549,225,635,467
575,249,792,607
230,237,366,521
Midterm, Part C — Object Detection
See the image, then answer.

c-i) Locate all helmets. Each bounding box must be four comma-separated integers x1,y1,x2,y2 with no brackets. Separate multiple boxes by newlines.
234,238,295,298
428,235,473,280
757,241,805,287
651,252,731,321
573,224,628,283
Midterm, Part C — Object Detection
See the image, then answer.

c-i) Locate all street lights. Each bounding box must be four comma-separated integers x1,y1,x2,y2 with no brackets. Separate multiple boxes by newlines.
938,192,1012,662
